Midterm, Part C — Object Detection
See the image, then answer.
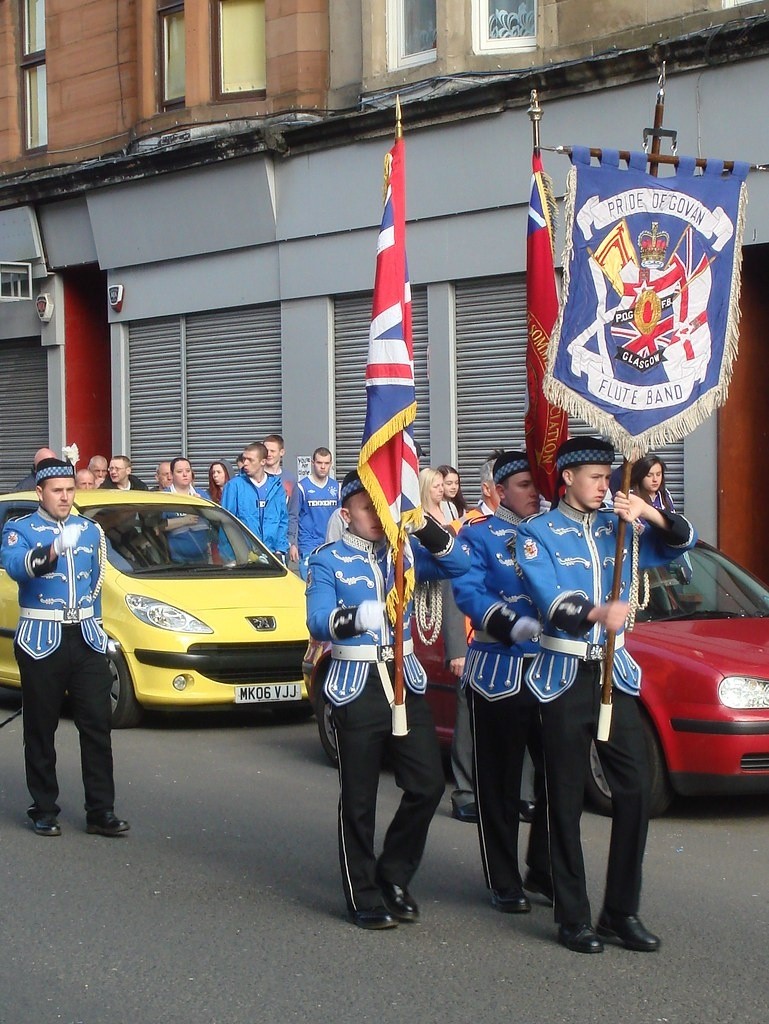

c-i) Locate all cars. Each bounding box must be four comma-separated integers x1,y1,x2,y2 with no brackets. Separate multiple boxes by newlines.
0,490,310,717
301,538,769,817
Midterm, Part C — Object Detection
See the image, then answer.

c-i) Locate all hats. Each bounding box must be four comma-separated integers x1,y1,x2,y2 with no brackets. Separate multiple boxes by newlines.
557,435,615,470
35,457,76,484
340,470,365,503
493,451,531,485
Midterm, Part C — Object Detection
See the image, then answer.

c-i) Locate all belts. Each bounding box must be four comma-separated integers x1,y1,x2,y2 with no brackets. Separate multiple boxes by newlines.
330,638,414,663
19,607,95,623
538,633,626,661
473,629,499,644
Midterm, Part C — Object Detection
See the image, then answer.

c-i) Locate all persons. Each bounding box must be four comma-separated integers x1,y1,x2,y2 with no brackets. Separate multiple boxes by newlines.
516,436,698,955
410,442,474,528
631,454,693,614
287,447,344,583
305,469,472,931
11,434,295,568
0,458,131,836
608,456,634,498
441,449,536,823
451,449,557,914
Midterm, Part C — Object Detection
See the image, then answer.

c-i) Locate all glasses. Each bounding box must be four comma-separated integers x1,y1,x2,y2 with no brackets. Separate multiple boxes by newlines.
107,466,130,472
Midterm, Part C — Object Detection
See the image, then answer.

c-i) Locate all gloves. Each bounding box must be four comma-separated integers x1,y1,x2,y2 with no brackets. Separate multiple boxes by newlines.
509,615,541,644
55,524,81,555
406,517,427,535
354,600,388,632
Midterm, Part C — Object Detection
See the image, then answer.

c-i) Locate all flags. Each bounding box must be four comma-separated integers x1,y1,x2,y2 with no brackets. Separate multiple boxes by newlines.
354,137,426,632
522,151,569,509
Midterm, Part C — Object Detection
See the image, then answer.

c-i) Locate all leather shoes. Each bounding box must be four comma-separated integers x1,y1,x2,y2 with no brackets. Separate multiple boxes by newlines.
556,919,604,952
595,909,660,951
375,879,419,920
523,873,554,902
34,817,61,836
489,887,532,912
346,907,398,929
86,811,129,834
519,798,536,820
451,802,477,823
